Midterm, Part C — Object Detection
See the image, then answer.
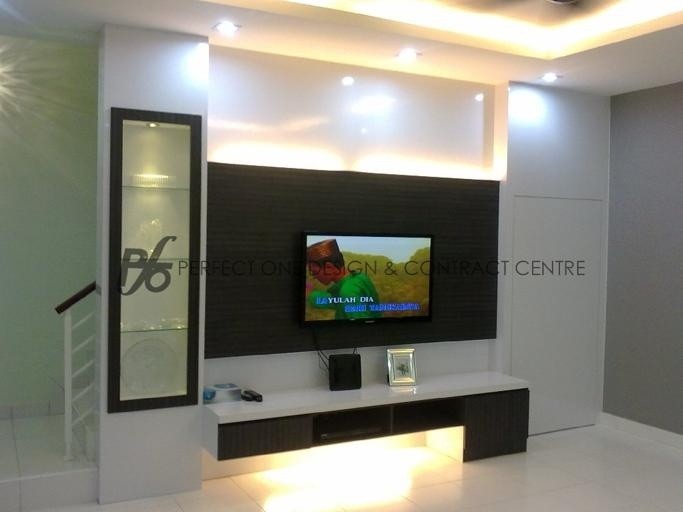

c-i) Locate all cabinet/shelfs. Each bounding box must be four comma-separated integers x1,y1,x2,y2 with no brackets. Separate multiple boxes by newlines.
201,389,529,463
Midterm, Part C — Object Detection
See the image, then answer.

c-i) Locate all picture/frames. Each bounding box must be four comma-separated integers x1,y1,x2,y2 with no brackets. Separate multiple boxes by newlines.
386,348,416,386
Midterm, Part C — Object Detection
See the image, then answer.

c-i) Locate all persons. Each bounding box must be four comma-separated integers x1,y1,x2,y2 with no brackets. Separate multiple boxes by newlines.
306,238,383,320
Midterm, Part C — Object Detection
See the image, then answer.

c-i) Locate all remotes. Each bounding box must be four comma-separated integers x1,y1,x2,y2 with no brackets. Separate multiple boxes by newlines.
244,390,264,402
241,393,252,401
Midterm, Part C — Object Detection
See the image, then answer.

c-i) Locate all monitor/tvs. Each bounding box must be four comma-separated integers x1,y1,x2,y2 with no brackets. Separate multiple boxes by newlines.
294,230,435,329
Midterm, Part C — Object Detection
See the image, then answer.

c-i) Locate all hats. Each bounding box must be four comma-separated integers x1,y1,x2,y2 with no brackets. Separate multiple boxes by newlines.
306,238,340,264
